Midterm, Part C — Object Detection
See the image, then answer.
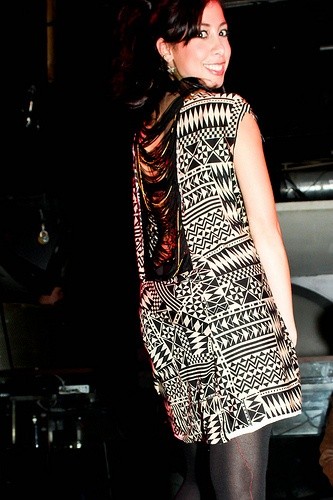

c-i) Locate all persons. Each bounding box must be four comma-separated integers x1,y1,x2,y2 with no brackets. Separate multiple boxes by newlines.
26,271,89,367
106,0,302,500
